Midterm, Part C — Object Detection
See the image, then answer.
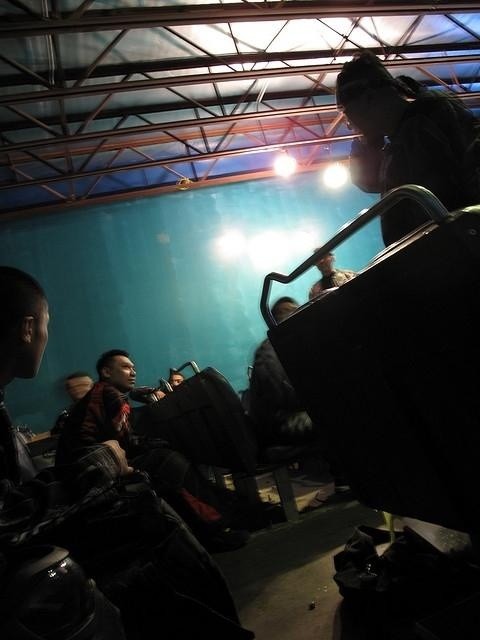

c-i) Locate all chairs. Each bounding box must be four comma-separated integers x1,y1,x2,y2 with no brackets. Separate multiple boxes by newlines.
0,183,479,639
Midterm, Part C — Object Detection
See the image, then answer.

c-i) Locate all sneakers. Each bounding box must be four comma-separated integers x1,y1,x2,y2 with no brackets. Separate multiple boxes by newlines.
205,527,251,556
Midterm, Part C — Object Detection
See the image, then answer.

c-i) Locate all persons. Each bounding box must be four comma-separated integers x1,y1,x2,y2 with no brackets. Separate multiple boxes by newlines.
76,350,222,525
253,297,312,438
168,372,185,387
332,47,480,249
308,246,357,302
51,373,93,429
1,265,254,640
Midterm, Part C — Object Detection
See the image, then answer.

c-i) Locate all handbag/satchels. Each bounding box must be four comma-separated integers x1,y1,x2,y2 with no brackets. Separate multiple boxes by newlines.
332,521,479,638
